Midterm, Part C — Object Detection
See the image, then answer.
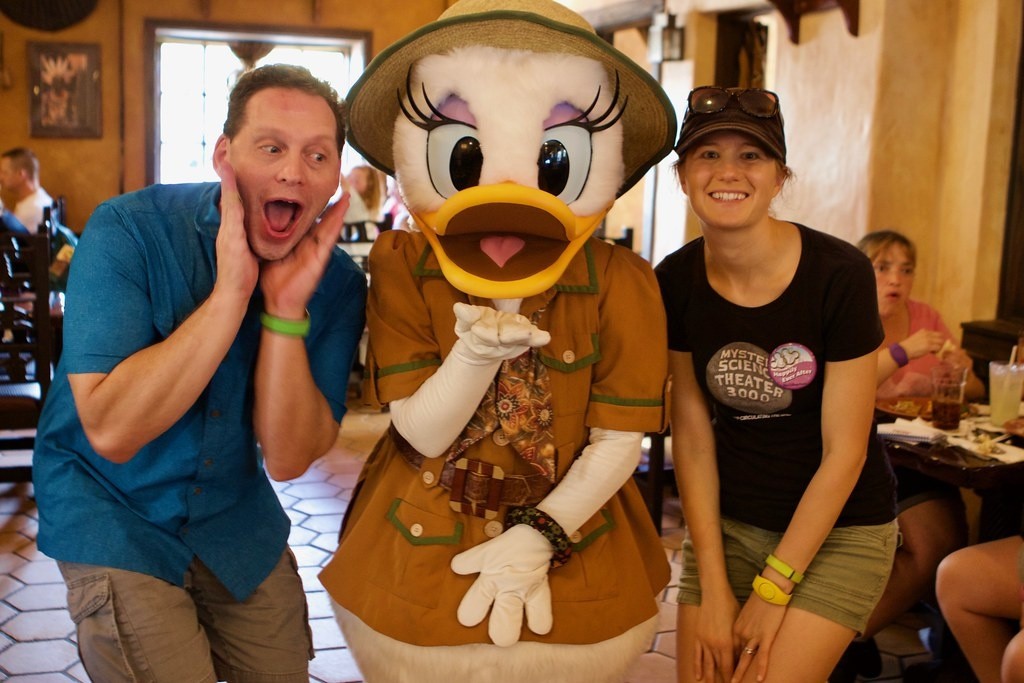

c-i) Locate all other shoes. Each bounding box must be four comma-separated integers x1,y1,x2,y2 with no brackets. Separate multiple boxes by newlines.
828,647,859,683
851,635,884,681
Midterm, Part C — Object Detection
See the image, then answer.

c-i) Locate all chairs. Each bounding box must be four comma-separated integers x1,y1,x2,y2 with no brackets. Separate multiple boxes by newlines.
0,194,67,482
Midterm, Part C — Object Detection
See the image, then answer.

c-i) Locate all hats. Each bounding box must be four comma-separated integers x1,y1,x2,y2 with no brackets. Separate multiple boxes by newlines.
674,88,787,165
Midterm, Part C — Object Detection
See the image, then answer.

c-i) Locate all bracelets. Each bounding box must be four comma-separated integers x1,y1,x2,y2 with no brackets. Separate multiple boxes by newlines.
888,343,908,367
505,505,572,570
261,309,310,336
766,554,804,583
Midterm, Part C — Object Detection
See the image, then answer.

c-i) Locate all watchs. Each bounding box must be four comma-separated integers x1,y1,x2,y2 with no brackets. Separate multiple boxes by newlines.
752,574,792,605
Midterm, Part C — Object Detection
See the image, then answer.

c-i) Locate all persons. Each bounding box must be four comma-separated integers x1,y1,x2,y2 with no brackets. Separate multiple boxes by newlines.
32,64,370,683
936,534,1024,683
655,86,896,683
325,166,414,380
0,148,55,384
859,230,986,683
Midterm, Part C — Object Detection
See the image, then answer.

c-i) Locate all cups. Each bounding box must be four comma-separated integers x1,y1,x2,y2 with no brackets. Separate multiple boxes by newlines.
930,365,967,431
989,361,1024,427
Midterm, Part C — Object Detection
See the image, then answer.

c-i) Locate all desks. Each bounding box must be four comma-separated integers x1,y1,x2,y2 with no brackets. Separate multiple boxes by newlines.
877,399,1024,549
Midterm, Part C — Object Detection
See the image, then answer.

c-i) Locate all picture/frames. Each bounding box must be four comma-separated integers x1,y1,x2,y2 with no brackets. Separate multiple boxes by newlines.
25,39,104,140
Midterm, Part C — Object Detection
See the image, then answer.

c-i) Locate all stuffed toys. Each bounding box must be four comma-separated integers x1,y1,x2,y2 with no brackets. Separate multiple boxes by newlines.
318,0,677,683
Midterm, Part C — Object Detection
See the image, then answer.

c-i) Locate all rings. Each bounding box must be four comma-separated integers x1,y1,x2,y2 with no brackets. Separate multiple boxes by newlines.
745,647,756,655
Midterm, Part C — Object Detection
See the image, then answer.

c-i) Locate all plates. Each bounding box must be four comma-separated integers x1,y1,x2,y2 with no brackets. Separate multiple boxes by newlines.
874,395,978,419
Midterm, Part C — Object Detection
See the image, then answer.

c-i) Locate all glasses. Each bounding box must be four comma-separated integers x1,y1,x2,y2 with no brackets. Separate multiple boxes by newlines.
679,86,785,135
